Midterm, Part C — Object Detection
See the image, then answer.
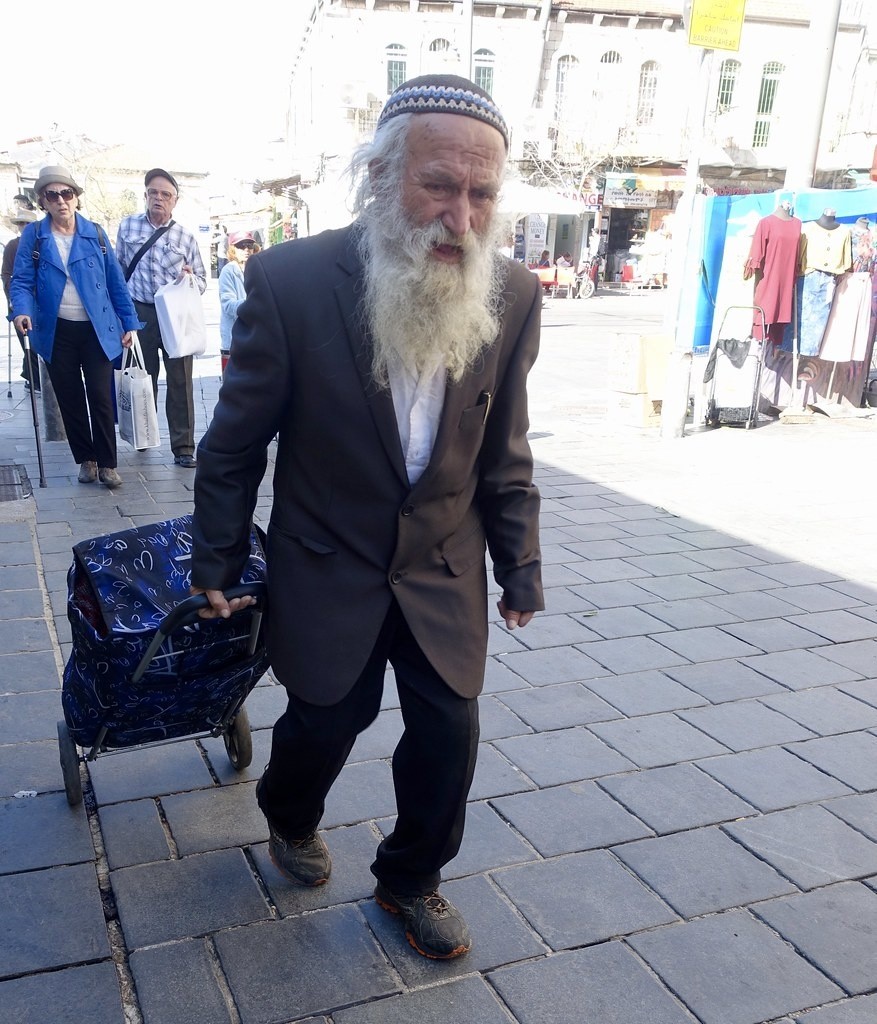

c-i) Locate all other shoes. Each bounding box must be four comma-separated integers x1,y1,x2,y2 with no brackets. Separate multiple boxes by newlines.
23,381,41,394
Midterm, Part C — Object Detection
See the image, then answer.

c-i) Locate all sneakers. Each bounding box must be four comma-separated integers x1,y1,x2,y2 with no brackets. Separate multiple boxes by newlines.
266,816,332,886
371,879,472,959
98,467,122,486
77,461,97,483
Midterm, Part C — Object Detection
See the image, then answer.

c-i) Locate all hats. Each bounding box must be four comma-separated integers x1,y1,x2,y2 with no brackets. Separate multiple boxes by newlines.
229,230,256,244
144,168,179,192
33,166,84,198
9,207,40,225
375,74,510,157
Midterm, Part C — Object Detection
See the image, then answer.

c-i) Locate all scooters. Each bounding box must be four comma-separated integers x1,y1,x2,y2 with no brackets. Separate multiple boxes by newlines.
571,255,602,299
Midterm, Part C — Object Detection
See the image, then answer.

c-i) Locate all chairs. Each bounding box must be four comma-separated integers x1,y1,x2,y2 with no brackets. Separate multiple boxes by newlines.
530,266,576,299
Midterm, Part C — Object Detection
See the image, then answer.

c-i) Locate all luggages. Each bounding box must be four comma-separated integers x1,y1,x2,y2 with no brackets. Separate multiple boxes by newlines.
54,513,272,806
703,306,766,430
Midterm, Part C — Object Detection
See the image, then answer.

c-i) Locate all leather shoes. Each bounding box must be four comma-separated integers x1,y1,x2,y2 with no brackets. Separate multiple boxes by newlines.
174,455,197,467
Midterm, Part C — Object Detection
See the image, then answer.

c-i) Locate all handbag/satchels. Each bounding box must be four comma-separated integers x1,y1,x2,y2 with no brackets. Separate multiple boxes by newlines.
153,271,205,359
113,331,162,450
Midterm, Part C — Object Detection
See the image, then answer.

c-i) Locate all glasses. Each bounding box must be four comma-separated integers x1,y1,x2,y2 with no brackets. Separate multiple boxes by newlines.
146,186,177,201
18,221,30,226
37,188,76,202
234,241,255,249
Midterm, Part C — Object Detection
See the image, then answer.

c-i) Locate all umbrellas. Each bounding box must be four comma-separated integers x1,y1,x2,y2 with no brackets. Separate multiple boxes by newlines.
492,178,587,262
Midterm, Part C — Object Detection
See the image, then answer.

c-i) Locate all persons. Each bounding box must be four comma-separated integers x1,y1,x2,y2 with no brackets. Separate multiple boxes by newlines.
538,250,552,294
189,75,547,960
819,217,877,362
588,227,601,291
114,168,208,467
0,206,42,394
777,208,853,356
5,165,149,488
217,225,229,276
219,231,262,372
556,252,573,267
749,202,802,323
